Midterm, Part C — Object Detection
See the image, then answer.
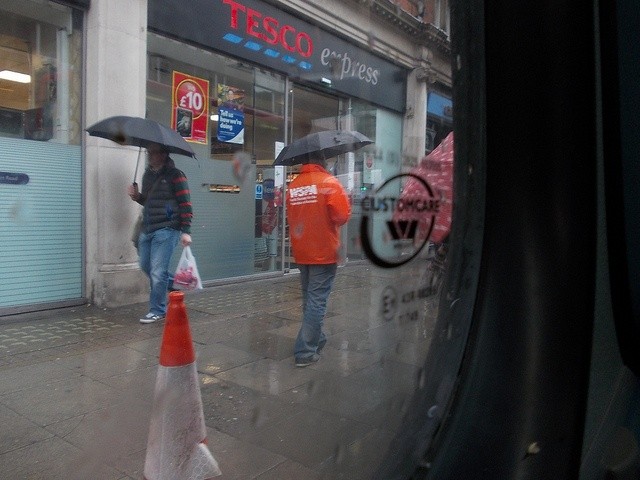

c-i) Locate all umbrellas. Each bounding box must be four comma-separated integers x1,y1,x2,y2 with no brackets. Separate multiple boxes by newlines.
85,116,201,186
272,130,375,166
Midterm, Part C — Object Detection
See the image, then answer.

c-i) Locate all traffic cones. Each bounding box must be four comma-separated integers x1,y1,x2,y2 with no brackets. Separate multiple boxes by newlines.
143,291,222,479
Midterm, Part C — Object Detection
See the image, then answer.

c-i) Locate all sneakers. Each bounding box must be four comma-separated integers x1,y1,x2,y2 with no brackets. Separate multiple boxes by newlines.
139,312,166,323
294,355,320,367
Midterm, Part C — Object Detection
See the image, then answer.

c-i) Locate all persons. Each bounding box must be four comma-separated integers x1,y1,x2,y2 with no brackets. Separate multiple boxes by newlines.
286,149,351,366
128,141,193,324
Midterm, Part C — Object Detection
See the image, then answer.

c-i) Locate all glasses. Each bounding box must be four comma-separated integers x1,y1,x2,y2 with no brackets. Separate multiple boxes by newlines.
144,149,166,156
